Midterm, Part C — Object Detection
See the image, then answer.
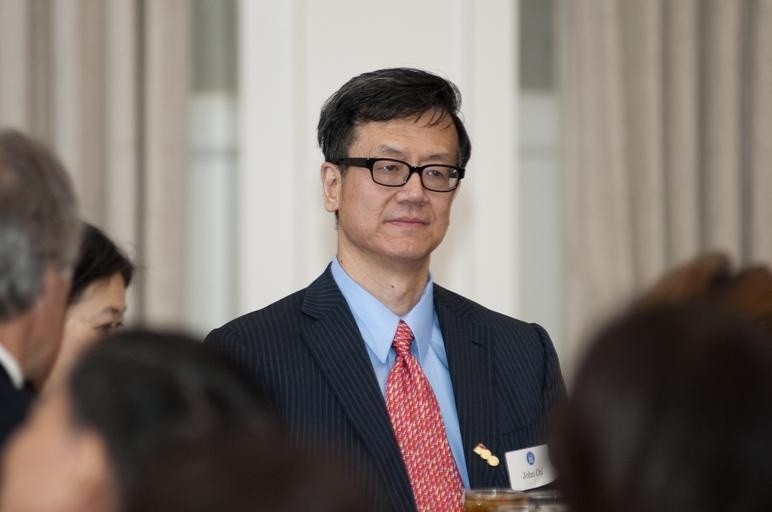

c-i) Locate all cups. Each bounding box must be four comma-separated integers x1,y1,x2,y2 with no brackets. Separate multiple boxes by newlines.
461,487,530,512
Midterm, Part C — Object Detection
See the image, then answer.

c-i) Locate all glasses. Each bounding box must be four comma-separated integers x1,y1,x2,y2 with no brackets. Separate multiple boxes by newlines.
336,157,465,192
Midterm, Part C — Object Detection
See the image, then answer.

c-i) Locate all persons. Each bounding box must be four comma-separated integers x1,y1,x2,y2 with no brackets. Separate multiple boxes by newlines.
548,251,771,512
201,66,570,512
1,325,280,512
40,219,137,400
1,125,86,443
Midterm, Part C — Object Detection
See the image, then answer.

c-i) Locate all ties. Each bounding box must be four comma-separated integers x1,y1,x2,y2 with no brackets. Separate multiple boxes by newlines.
386,320,465,512
